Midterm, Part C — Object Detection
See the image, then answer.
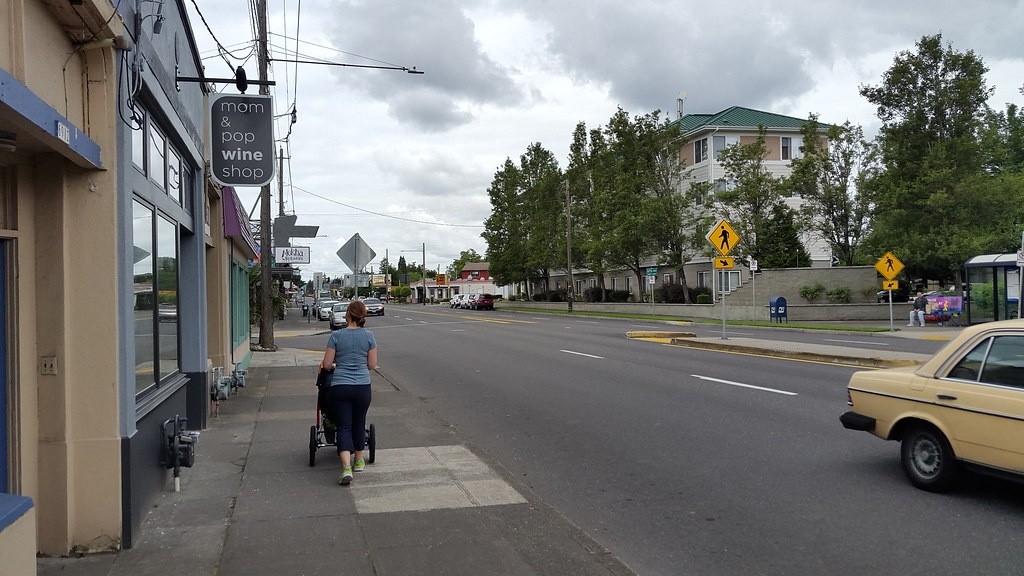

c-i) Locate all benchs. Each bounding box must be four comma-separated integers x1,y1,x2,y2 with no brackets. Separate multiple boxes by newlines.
917,296,963,328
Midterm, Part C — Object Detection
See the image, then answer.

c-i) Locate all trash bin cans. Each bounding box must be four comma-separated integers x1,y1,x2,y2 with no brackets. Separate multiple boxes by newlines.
302,305,309,317
770,297,788,323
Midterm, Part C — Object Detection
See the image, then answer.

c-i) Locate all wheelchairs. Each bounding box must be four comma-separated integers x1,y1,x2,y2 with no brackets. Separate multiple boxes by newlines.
309,360,379,467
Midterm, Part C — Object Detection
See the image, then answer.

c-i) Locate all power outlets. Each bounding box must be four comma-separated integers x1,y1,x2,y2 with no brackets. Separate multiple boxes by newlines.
40,356,58,375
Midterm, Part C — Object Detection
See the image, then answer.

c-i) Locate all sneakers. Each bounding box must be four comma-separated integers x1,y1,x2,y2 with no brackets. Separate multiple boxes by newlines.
337,468,353,485
352,457,365,471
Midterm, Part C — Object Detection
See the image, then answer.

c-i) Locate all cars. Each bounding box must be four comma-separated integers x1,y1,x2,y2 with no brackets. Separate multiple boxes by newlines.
329,301,352,330
839,318,1024,492
311,290,387,322
362,297,385,316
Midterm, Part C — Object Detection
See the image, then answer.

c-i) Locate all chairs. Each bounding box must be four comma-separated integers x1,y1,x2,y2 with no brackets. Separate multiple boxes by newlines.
1010,311,1024,319
956,362,1004,386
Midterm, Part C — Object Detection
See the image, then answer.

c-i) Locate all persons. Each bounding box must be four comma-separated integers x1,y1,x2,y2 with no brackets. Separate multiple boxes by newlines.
907,292,928,326
323,301,377,485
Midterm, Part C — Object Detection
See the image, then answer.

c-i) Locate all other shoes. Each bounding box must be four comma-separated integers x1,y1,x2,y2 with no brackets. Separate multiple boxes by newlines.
907,323,914,326
921,324,925,327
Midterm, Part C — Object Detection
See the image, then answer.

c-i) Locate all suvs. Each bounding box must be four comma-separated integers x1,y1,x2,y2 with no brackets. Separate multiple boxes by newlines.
470,293,494,311
449,294,465,308
459,293,476,310
